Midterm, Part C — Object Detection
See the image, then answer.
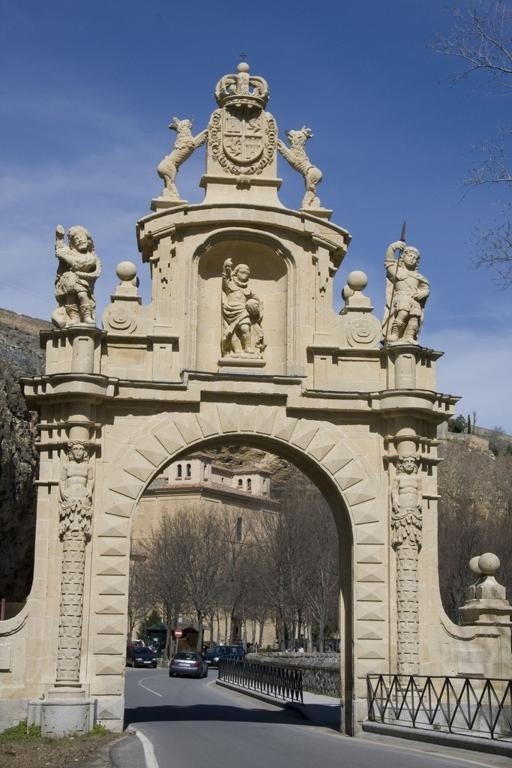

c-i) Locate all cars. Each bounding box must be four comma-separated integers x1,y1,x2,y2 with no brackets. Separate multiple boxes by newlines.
126,639,158,668
168,650,209,678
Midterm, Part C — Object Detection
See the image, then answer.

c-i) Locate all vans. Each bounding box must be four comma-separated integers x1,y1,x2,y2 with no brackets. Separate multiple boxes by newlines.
203,645,247,668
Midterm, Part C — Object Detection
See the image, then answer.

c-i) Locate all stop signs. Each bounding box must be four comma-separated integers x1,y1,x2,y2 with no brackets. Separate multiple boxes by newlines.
174,628,182,637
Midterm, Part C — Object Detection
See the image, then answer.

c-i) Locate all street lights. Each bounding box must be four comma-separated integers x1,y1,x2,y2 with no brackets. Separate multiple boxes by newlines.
300,634,305,648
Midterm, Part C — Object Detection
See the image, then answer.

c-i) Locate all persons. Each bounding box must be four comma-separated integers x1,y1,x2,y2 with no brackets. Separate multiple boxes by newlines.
59,440,95,502
221,257,260,354
51,224,102,327
392,453,423,516
384,241,431,345
